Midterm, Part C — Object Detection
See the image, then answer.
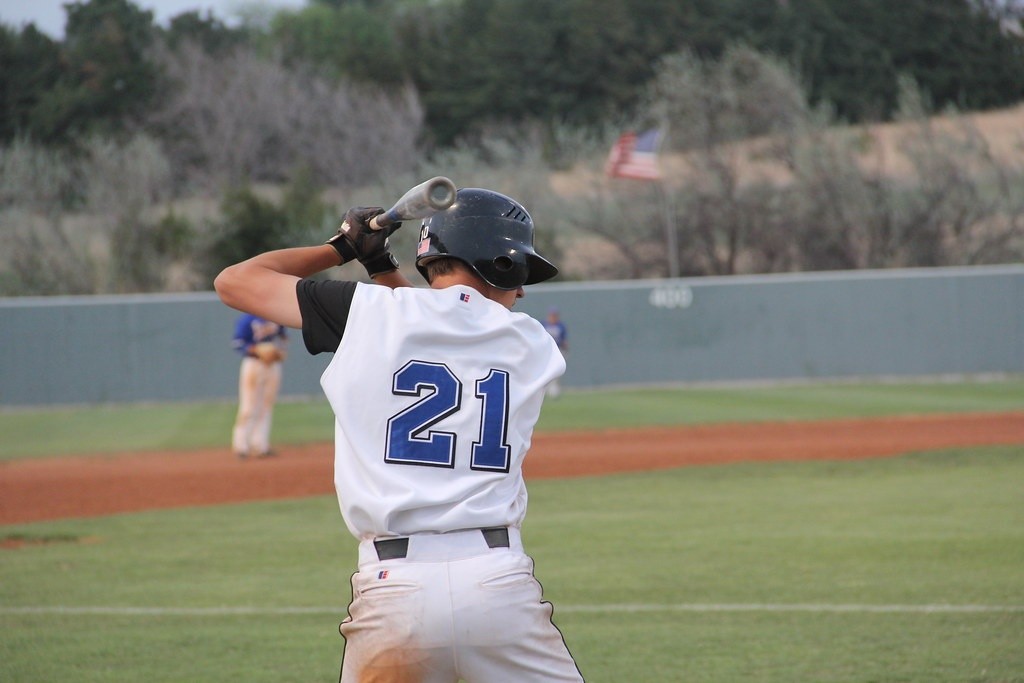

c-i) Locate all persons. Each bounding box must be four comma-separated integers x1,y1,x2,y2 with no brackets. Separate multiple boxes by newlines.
540,308,569,400
231,313,290,459
214,188,586,683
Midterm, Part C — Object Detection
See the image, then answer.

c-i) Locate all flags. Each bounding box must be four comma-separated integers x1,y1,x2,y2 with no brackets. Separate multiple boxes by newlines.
605,126,666,181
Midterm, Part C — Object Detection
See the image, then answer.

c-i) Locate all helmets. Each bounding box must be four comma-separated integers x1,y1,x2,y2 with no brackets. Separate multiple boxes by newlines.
416,187,560,290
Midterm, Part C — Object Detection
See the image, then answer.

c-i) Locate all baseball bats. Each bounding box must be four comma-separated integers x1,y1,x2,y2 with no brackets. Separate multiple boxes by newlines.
369,175,458,230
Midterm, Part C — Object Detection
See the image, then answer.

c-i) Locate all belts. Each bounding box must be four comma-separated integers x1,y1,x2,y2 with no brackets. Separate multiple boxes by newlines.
374,527,509,560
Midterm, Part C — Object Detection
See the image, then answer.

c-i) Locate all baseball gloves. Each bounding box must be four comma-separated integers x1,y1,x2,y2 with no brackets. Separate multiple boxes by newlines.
256,342,277,363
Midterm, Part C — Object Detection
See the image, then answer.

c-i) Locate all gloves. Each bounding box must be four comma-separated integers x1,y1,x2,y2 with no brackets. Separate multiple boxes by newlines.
325,206,402,277
